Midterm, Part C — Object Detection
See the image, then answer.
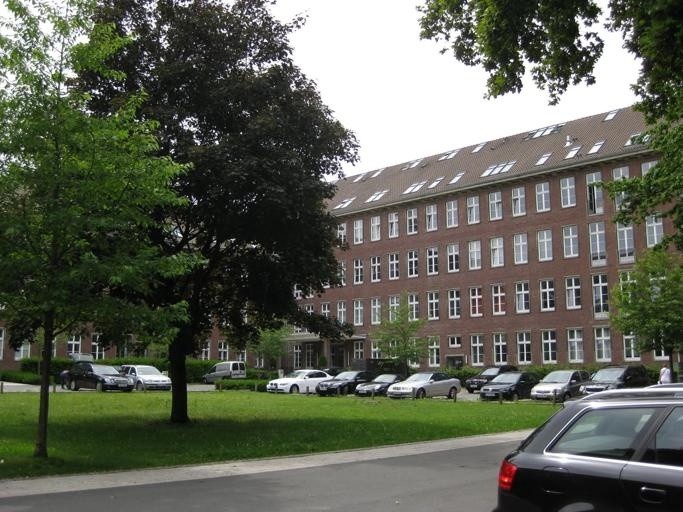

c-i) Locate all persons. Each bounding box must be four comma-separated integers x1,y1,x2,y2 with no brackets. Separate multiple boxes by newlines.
657,362,672,384
58,370,70,388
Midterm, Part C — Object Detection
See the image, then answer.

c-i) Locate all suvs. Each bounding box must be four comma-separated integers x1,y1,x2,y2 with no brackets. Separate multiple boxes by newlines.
578,364,651,396
492,384,683,511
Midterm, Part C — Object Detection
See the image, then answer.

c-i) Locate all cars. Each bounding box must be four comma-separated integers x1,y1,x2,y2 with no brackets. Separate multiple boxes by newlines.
530,370,589,401
266,365,462,399
463,365,520,393
121,364,171,391
65,363,134,391
479,372,538,402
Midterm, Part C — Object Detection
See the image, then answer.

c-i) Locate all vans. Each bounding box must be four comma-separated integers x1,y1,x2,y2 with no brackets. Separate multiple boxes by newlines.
201,361,246,384
